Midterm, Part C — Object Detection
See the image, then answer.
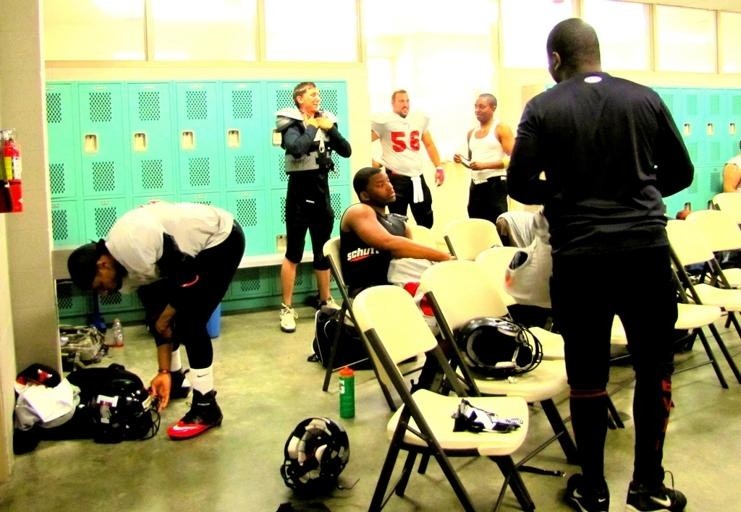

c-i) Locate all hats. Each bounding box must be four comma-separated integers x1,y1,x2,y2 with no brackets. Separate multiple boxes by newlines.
68,244,98,291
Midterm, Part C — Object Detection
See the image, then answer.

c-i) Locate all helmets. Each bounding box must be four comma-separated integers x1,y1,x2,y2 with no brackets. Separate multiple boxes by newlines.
88,379,161,443
459,317,543,380
280,417,350,491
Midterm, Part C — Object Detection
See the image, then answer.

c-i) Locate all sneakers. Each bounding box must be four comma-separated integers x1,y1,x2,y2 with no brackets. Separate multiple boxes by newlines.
280,302,299,332
316,295,352,318
168,391,223,438
567,472,610,511
148,369,191,398
627,470,687,511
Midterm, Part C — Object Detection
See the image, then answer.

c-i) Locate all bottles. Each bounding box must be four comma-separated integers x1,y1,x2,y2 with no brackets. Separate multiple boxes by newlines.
114,319,123,345
338,366,355,418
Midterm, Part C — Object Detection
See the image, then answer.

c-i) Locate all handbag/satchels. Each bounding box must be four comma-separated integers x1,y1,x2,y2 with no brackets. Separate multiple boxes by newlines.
59,324,109,363
308,306,375,370
67,363,144,405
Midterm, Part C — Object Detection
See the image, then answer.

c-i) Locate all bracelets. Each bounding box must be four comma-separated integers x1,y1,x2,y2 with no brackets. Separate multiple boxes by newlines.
157,367,172,375
435,164,445,171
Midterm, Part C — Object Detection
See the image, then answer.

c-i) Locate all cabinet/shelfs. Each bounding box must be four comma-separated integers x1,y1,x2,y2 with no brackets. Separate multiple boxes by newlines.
643,87,741,220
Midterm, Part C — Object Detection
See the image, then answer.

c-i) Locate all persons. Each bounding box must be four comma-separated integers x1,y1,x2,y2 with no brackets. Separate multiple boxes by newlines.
273,82,351,334
721,155,741,192
67,201,246,441
370,88,445,230
335,166,459,330
503,18,697,511
453,93,515,223
